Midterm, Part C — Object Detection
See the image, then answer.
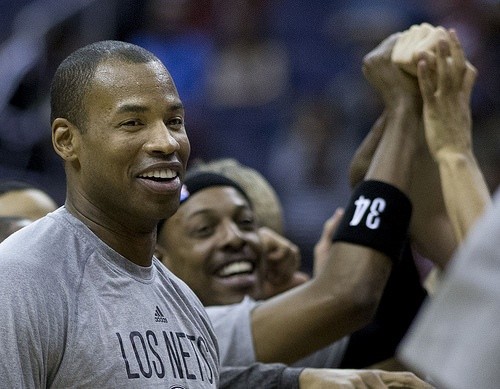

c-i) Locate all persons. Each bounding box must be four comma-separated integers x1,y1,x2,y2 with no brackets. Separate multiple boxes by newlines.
154,18,500,389
1,178,58,242
0,42,220,389
